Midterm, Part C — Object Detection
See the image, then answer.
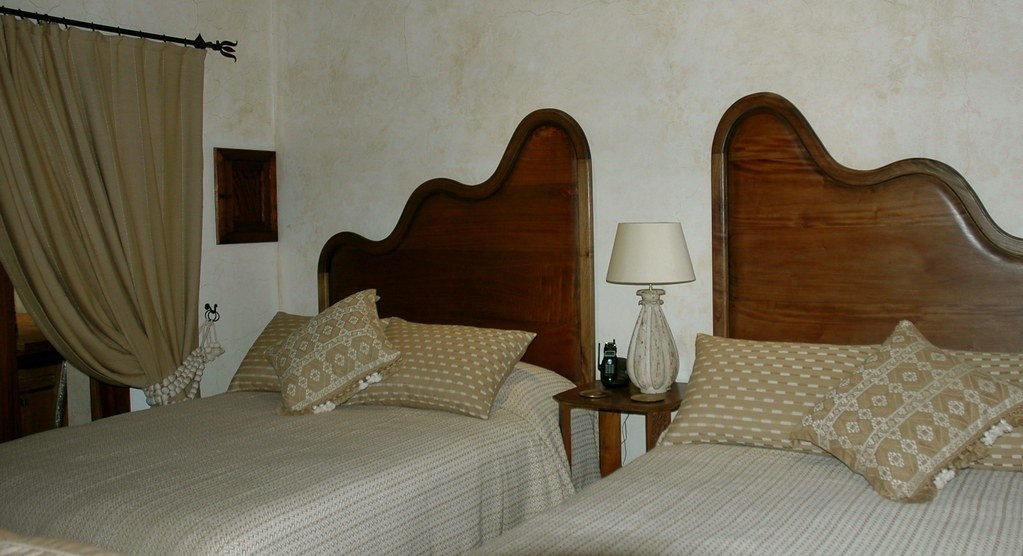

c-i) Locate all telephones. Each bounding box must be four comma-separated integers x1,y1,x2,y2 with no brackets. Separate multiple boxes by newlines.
598,338,627,388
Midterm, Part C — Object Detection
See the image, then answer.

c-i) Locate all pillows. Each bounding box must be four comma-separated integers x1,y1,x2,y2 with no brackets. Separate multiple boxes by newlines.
654,333,881,451
789,319,1023,501
224,310,314,392
261,289,402,416
342,317,536,420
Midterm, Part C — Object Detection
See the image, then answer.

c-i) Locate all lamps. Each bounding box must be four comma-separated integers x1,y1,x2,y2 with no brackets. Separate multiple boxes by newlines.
605,221,695,394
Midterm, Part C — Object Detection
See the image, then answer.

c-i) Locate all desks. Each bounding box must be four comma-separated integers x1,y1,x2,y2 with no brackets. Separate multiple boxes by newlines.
553,380,688,478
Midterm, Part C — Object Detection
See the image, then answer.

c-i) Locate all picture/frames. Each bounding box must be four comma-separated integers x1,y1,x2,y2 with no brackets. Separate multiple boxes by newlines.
214,147,279,246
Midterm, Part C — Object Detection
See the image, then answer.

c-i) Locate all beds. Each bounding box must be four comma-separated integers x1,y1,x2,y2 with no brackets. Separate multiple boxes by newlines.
0,109,601,556
461,90,1023,556
